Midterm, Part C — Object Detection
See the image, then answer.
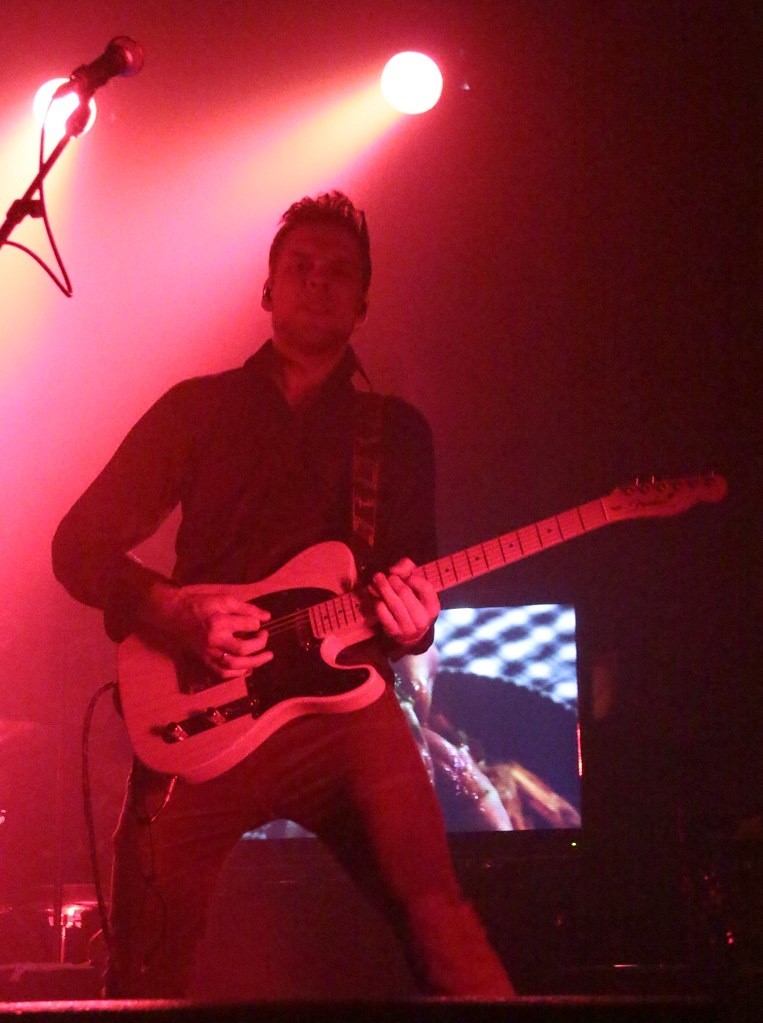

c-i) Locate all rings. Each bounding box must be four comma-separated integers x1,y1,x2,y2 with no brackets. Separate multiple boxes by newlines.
218,652,228,665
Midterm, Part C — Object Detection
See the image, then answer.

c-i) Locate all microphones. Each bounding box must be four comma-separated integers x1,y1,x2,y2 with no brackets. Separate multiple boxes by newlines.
51,34,145,104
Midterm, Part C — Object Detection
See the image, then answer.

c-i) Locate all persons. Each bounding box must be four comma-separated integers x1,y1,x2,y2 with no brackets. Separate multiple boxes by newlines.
51,190,516,1004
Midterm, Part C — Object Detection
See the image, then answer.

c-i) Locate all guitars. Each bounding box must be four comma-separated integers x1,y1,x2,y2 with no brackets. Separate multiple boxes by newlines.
111,468,726,785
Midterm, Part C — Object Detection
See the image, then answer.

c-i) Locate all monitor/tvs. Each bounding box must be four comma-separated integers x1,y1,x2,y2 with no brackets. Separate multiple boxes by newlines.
227,585,593,870
